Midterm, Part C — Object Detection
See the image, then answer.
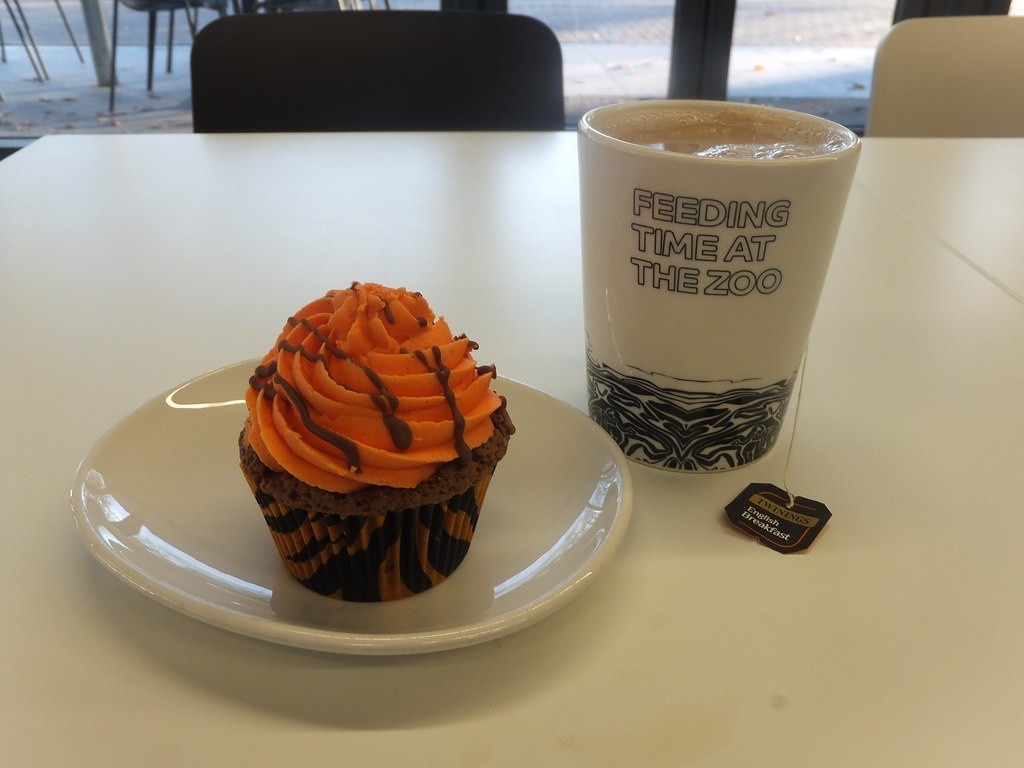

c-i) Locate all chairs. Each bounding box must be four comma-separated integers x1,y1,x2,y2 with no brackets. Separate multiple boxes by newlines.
0,0,1024,133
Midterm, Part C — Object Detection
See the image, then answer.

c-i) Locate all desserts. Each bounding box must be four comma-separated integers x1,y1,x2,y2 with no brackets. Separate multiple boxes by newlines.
237,278,517,604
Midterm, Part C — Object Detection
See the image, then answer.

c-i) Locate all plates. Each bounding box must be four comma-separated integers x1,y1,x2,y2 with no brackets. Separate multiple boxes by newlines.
70,357,633,656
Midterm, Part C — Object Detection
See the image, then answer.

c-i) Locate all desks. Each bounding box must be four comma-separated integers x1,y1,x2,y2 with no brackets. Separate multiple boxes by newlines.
0,131,1024,768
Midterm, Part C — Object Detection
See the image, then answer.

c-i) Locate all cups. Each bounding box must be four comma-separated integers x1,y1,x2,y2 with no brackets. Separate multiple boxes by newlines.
577,99,862,475
